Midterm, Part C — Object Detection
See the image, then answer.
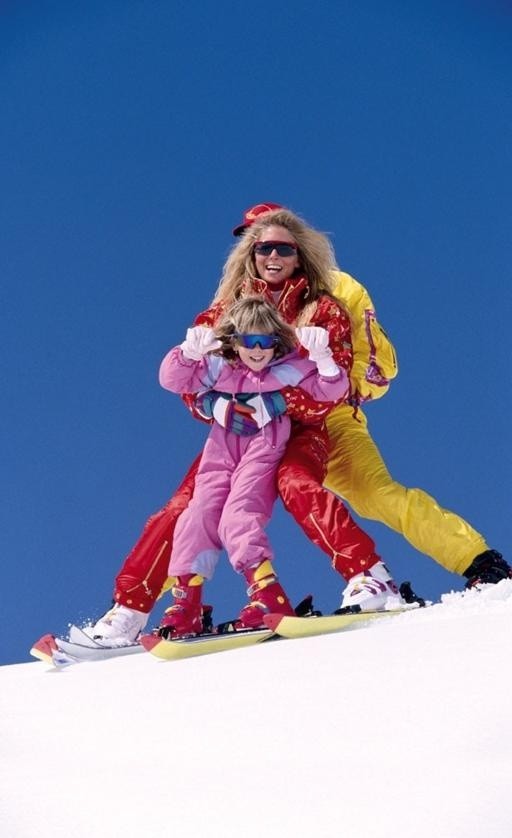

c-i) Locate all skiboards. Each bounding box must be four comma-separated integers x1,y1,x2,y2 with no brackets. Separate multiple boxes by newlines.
31,610,407,669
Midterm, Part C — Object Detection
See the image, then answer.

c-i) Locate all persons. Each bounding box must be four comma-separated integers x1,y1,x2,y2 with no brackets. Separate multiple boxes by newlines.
158,295,350,639
91,209,406,643
233,204,512,591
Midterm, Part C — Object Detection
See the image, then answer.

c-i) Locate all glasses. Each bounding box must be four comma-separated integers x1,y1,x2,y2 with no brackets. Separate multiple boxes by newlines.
232,334,280,349
252,242,296,256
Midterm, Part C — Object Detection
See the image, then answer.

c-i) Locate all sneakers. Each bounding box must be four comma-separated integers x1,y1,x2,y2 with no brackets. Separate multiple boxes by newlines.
463,549,511,588
93,602,151,647
340,561,402,610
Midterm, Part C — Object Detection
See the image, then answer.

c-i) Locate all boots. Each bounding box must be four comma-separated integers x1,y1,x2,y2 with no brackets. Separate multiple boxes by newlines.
240,557,296,627
161,573,205,635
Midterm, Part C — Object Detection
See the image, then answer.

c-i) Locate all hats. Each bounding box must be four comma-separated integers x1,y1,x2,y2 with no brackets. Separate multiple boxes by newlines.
233,205,289,236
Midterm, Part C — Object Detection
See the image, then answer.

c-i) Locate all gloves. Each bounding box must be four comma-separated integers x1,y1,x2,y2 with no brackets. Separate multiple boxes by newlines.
180,327,222,362
295,326,340,377
193,390,286,437
